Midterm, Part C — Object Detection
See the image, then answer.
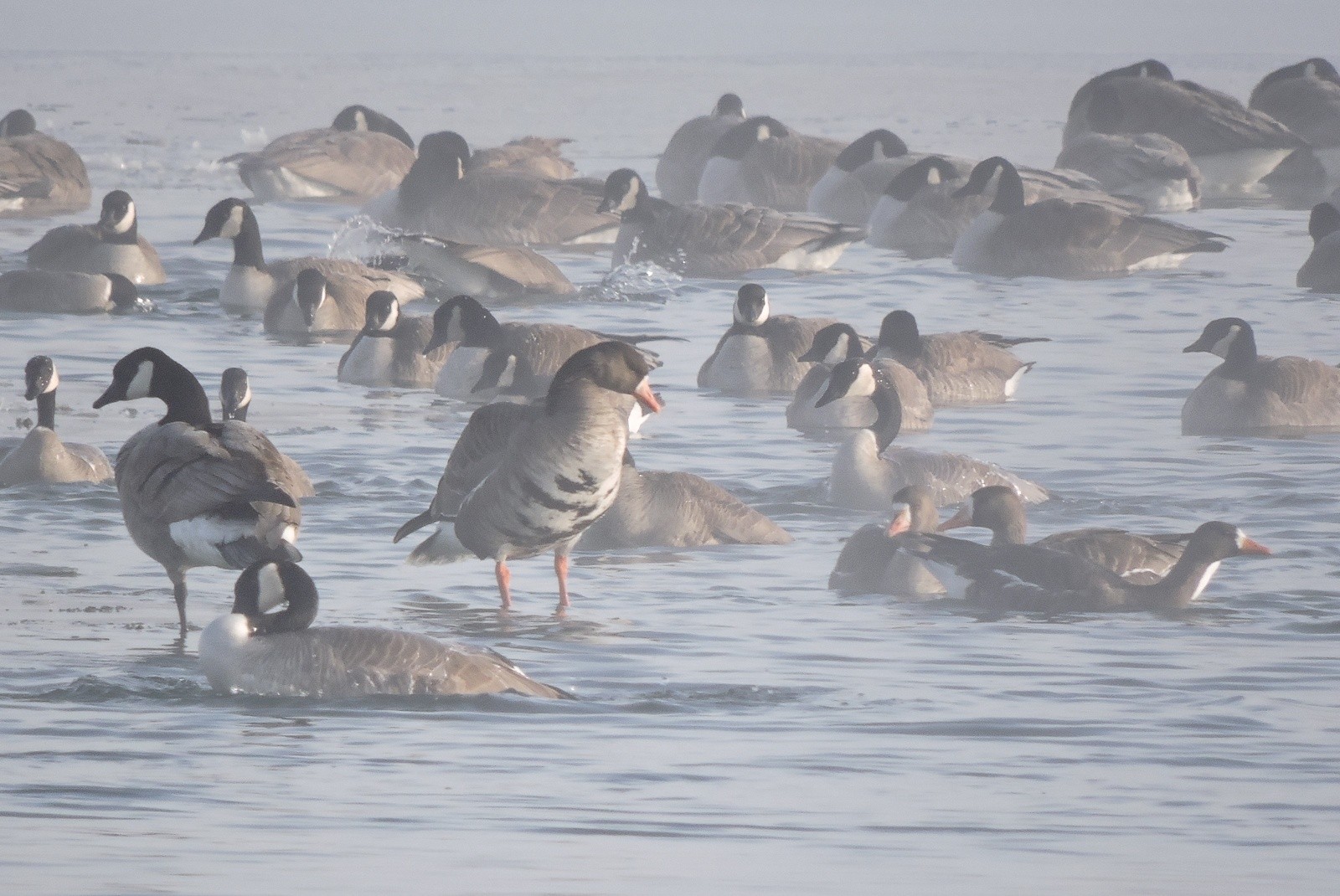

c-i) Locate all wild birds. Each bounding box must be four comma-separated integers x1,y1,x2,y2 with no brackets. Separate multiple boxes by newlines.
593,58,1340,293
199,558,581,703
696,283,1339,614
1,103,800,639
